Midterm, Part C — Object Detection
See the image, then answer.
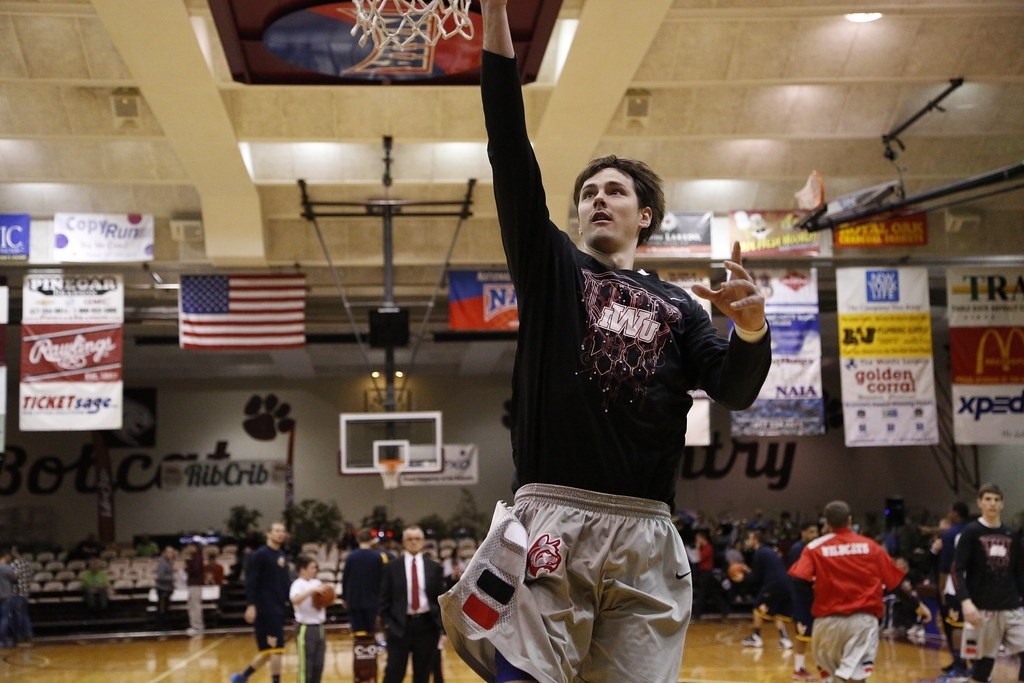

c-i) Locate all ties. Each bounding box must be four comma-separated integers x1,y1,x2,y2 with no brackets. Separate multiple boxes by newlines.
412,558,419,611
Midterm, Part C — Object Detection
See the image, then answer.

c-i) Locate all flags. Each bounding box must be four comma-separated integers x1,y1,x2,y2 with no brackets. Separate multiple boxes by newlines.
178,274,307,350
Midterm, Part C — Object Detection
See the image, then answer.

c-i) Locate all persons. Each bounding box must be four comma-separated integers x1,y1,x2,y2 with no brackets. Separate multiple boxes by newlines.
155,521,486,637
287,557,336,683
788,500,908,683
437,1,771,683
951,482,1024,683
372,525,446,683
931,501,971,683
65,533,159,617
341,528,384,641
788,523,834,683
0,544,35,649
670,498,1024,646
233,522,292,683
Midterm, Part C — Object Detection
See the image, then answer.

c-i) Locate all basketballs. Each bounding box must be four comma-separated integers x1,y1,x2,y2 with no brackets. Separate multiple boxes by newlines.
312,584,335,607
728,563,744,578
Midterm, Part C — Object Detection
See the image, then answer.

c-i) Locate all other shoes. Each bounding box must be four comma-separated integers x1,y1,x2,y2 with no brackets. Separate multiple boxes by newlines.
776,639,793,649
231,675,245,683
740,634,762,647
792,669,816,679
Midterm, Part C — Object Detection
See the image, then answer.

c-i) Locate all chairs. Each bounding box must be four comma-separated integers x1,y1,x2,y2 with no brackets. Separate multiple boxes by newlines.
18,539,486,619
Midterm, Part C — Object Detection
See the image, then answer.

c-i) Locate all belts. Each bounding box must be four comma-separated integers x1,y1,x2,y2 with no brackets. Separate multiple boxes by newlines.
407,612,430,618
